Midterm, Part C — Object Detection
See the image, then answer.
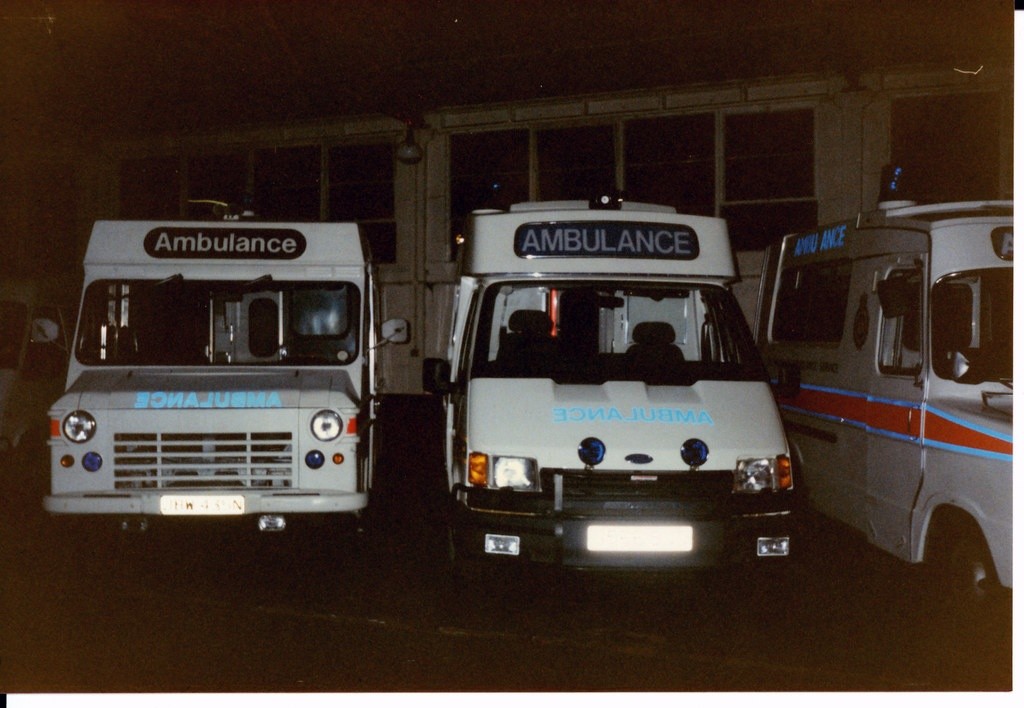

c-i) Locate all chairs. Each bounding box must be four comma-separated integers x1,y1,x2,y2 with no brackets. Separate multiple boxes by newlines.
626,322,687,361
492,311,568,380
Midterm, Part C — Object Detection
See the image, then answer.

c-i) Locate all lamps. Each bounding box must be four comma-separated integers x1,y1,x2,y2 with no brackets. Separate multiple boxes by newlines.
396,124,424,164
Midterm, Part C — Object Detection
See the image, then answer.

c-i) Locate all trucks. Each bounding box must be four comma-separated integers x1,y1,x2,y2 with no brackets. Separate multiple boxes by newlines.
420,197,804,590
752,197,1012,633
41,216,410,553
